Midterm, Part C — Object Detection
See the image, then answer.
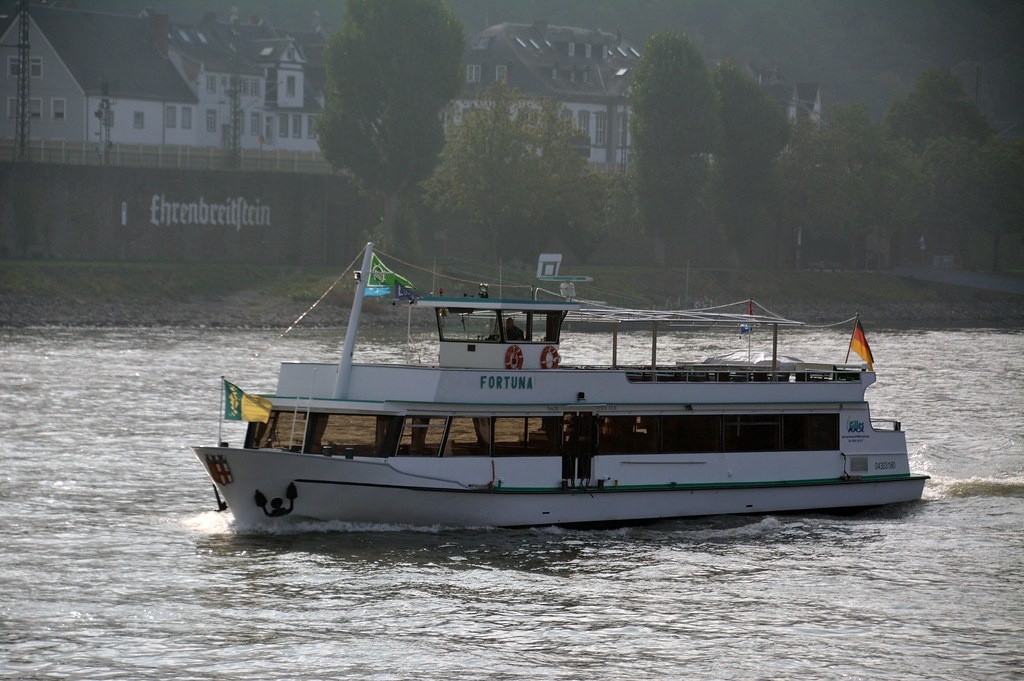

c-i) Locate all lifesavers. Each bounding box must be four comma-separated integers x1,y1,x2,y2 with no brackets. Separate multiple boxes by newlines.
505,345,524,369
541,346,558,369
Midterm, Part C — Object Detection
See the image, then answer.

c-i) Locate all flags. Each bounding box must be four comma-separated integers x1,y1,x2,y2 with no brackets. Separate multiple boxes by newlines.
367,253,421,305
223,380,273,424
850,316,875,373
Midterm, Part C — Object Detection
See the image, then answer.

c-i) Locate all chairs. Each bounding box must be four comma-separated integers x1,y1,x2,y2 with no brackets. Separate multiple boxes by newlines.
643,365,810,381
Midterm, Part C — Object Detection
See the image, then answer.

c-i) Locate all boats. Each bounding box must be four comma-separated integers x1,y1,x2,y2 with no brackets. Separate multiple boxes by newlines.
190,243,931,532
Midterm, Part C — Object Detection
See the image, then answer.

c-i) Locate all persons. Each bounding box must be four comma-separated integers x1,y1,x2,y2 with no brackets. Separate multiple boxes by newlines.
506,318,523,341
